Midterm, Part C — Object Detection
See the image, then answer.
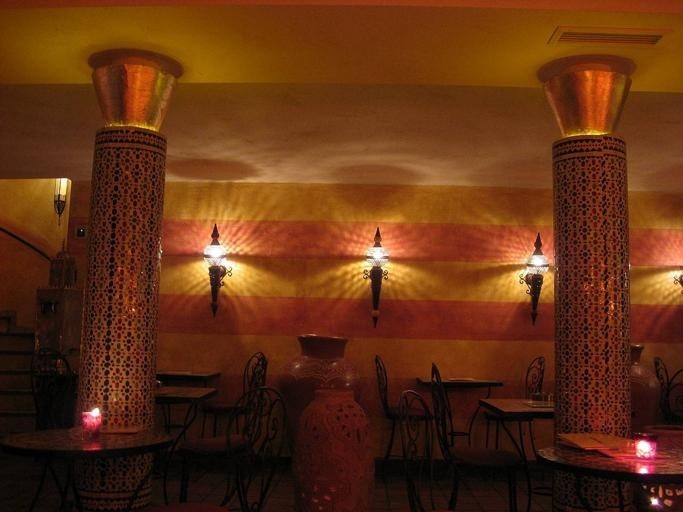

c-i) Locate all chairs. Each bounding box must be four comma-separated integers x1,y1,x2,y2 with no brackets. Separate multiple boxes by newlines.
375,355,525,512
142,350,288,512
30,348,86,511
652,357,682,425
483,358,545,486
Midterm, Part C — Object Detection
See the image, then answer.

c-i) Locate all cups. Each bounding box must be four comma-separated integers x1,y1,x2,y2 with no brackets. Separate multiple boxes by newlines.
634,432,658,460
82,412,103,438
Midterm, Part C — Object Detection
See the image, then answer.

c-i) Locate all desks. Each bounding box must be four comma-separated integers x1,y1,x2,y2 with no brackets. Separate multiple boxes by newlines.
481,399,558,512
7,427,172,457
538,438,682,484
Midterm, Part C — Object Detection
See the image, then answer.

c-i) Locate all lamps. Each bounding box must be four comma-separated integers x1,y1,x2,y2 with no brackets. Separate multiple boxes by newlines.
363,224,389,328
518,230,549,324
205,222,233,319
54,178,69,227
